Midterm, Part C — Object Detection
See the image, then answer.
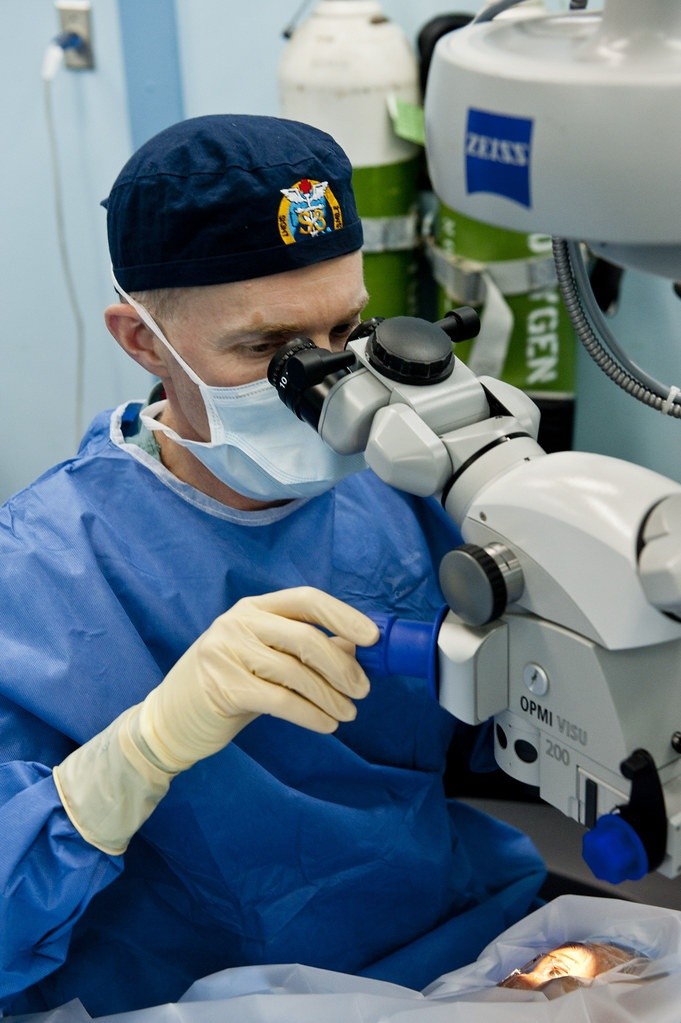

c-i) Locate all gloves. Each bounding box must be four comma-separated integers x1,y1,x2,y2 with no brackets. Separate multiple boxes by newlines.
53,585,380,856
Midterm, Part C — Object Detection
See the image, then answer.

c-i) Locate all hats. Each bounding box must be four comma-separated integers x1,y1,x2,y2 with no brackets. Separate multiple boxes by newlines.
100,114,364,295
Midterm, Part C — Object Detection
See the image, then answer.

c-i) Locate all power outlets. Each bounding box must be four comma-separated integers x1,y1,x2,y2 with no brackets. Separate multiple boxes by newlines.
60,5,94,69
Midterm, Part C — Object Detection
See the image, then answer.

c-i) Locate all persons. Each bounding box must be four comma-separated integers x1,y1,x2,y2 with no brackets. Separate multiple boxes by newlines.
0,113,623,1023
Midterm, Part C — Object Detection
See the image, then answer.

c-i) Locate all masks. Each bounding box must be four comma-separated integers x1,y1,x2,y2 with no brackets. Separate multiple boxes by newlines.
107,267,369,502
